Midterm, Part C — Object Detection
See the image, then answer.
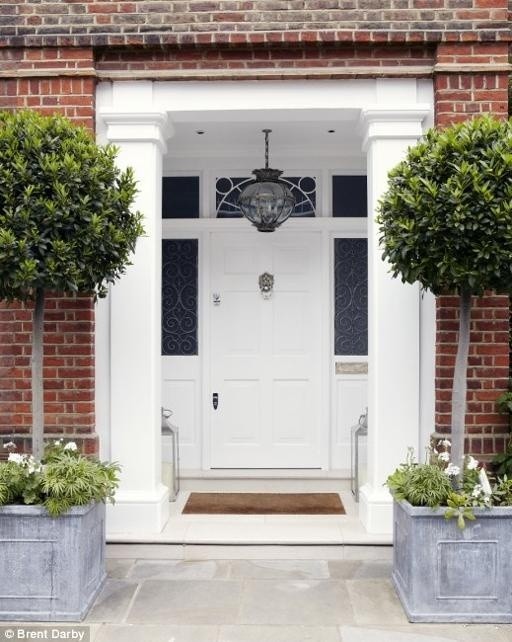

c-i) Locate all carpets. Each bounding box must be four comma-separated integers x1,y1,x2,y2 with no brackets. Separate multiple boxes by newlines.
180,490,348,516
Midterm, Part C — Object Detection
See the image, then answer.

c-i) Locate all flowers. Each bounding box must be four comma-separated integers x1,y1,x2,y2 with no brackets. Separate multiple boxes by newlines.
382,434,512,528
1,437,121,516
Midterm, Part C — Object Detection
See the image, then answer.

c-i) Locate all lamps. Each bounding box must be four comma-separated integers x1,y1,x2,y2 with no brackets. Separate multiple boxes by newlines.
236,129,296,233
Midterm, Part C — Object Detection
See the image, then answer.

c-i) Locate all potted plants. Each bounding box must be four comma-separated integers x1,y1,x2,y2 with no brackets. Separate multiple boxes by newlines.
0,109,148,625
372,112,512,626
